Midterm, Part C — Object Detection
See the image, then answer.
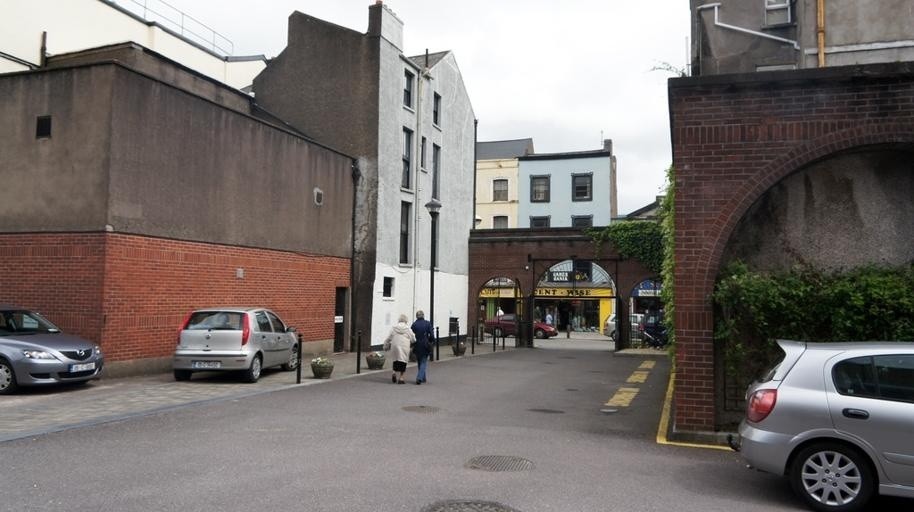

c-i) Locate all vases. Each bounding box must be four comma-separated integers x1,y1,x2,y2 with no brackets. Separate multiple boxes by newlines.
452,343,466,356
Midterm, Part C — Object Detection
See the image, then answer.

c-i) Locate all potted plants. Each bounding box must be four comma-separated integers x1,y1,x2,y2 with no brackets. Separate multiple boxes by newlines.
311,358,334,379
366,351,386,370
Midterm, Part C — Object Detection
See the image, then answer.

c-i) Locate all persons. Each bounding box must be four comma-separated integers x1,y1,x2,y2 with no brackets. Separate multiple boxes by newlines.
533,305,542,323
384,311,435,384
496,306,504,316
545,311,552,324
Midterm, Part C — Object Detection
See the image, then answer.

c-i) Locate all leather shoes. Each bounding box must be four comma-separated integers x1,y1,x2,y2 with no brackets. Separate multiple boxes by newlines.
415,378,426,384
392,374,397,383
398,380,405,384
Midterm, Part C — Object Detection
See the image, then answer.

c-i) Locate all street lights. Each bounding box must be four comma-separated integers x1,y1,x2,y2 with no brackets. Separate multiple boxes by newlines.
424,198,442,360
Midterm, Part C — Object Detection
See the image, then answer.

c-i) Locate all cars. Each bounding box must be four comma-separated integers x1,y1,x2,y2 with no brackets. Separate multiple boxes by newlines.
172,306,301,384
603,312,645,343
0,306,105,396
726,337,914,512
484,313,558,339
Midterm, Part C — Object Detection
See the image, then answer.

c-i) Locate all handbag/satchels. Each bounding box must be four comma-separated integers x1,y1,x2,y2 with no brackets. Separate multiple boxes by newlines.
424,337,433,354
